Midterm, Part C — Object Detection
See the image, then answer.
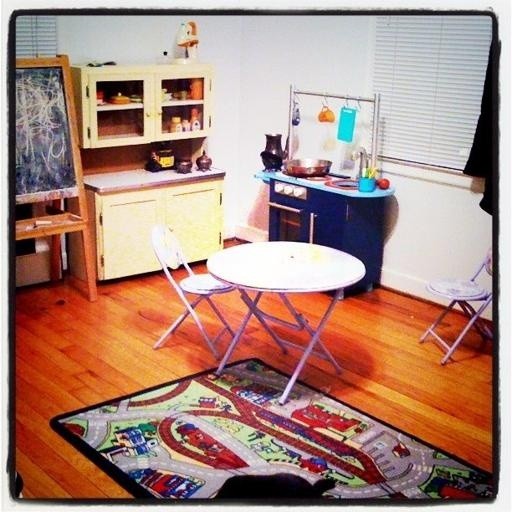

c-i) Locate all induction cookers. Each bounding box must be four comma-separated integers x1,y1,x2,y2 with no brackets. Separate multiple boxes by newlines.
269,172,346,203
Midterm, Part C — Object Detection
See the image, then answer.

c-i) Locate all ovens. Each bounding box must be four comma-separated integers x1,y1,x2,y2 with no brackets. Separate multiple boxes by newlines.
266,202,309,247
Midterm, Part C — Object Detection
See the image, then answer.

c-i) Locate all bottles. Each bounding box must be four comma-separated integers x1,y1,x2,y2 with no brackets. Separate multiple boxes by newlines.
170,107,201,132
177,150,214,172
176,22,187,58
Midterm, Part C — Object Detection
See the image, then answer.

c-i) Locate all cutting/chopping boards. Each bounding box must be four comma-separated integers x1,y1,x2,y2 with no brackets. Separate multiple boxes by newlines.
336,106,357,143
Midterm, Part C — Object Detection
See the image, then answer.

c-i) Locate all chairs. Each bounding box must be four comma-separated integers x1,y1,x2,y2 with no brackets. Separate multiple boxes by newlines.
419,244,492,366
151,224,237,360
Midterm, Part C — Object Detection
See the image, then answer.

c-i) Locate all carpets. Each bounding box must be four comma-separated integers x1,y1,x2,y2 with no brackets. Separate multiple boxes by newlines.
50,357,494,500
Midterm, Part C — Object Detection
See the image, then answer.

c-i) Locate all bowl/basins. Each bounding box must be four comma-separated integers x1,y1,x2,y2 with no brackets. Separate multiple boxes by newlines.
283,157,332,177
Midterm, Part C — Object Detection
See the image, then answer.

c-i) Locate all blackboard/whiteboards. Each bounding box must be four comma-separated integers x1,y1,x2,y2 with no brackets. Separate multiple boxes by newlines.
15,57,84,205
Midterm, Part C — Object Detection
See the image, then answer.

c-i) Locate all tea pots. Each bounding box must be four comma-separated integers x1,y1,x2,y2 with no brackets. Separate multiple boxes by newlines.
261,131,286,172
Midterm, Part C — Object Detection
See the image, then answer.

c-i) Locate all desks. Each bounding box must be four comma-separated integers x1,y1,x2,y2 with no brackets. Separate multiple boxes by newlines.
207,241,367,406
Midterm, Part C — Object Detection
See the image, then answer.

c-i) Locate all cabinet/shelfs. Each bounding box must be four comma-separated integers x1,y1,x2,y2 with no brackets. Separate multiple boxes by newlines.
71,62,215,149
68,162,226,282
